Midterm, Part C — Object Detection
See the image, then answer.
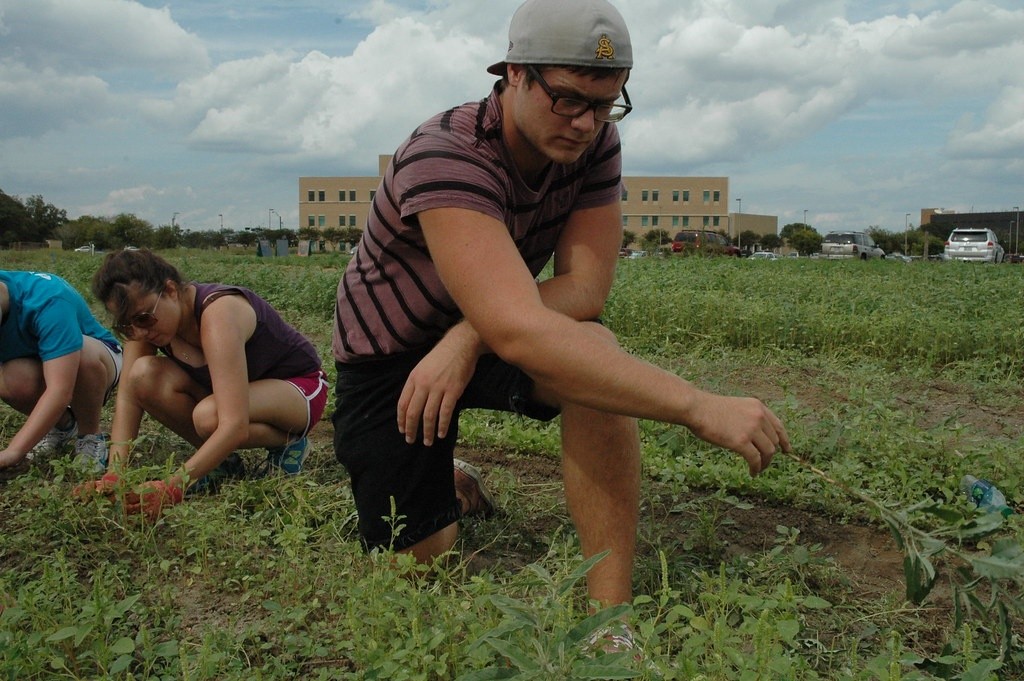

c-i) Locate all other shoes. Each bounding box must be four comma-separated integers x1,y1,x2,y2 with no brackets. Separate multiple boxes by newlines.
453,458,496,515
582,623,644,673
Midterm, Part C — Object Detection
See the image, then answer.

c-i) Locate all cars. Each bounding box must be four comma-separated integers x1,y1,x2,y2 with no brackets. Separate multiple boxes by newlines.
886,252,912,265
618,247,664,260
788,251,819,259
748,252,779,262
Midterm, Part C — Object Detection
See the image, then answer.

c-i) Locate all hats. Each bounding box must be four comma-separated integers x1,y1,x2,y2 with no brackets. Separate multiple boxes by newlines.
487,0,632,76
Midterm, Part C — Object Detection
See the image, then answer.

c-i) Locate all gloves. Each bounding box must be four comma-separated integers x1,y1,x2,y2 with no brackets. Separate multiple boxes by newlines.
73,472,125,504
115,475,182,521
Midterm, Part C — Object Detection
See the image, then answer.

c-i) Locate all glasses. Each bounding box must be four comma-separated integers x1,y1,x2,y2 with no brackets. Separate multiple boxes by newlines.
528,64,633,122
111,291,163,336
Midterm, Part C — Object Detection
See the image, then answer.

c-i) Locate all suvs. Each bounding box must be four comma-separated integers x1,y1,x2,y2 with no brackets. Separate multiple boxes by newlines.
673,229,741,260
942,226,1005,265
820,230,885,262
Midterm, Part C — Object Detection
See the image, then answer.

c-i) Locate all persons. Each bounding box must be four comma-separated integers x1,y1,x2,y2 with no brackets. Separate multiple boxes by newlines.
73,249,330,525
0,269,124,480
331,0,790,681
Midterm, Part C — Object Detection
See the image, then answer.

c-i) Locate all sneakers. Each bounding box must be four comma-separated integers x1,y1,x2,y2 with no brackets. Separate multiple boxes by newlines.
73,431,108,472
260,437,311,477
25,405,79,460
183,452,245,497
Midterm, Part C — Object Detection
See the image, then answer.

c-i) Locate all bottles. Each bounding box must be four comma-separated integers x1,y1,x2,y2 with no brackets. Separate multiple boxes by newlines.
959,474,1011,517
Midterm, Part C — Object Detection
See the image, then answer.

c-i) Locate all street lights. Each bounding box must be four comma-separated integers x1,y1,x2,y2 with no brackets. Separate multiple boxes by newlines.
905,214,911,256
1009,220,1016,255
803,209,809,230
736,198,741,247
1013,206,1020,254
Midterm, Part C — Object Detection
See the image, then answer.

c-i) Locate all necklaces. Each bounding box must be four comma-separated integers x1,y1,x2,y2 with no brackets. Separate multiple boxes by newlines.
173,303,191,360
